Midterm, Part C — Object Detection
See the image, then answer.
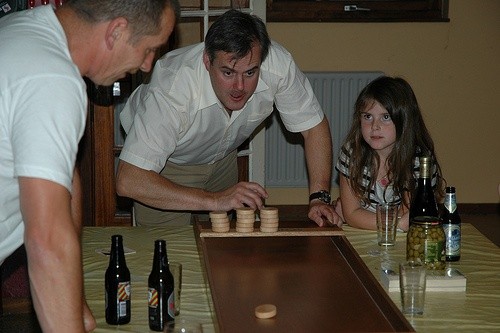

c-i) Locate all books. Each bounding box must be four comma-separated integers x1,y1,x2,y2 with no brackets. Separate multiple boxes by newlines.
386,286,466,293
382,269,466,288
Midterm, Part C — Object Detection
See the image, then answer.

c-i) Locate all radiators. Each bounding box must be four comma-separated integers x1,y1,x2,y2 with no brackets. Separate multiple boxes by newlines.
264,71,385,187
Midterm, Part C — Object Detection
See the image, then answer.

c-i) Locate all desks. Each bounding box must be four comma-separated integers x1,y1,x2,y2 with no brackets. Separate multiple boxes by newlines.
83,222,500,333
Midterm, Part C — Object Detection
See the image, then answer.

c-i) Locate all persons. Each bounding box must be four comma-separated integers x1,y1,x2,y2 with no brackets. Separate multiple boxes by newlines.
333,77,441,232
115,10,343,226
0,1,180,333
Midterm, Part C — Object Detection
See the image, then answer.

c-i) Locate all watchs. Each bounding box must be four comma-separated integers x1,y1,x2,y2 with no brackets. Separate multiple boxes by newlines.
309,190,331,203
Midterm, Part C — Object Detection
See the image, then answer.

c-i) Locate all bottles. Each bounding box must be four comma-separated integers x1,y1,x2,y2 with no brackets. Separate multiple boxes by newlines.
440,187,461,262
105,234,131,326
409,156,437,228
147,239,176,332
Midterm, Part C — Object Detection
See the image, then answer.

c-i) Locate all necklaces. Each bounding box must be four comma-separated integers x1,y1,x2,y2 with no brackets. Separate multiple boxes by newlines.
378,175,387,186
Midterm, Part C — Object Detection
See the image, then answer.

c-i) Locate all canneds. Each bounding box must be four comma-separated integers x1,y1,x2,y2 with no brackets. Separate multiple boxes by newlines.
406,217,446,271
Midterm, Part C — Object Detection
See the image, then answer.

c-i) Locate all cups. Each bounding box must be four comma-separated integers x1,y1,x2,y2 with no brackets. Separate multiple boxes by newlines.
376,202,400,247
168,261,181,316
398,260,427,316
